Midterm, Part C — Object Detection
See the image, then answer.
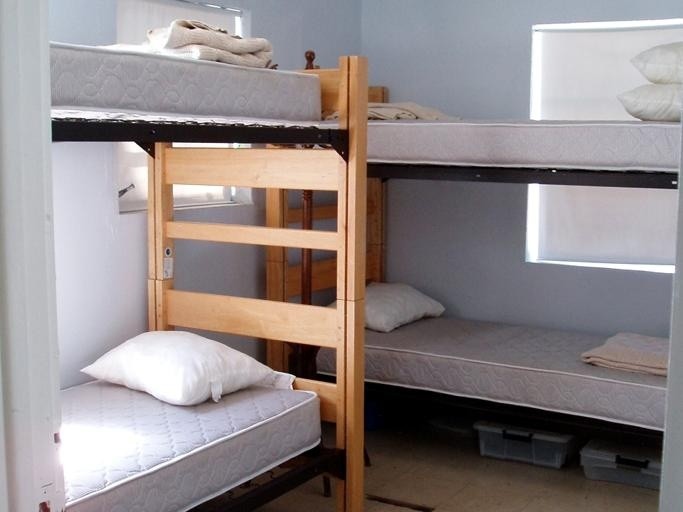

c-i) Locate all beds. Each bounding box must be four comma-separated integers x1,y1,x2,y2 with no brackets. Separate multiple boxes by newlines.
47,41,369,512
260,75,683,425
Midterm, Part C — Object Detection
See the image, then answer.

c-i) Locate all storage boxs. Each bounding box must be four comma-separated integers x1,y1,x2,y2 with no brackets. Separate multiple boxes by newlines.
580,436,663,490
474,414,582,469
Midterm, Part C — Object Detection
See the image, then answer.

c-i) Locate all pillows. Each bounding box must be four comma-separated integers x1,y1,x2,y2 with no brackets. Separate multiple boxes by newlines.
79,330,276,406
617,82,683,124
631,41,683,84
323,281,445,333
